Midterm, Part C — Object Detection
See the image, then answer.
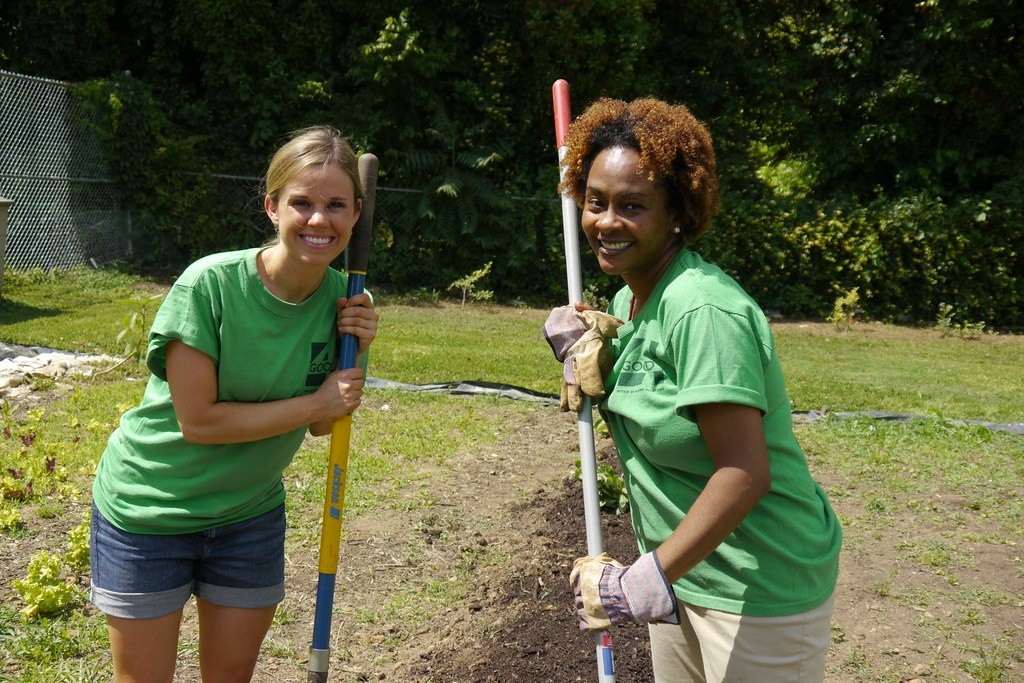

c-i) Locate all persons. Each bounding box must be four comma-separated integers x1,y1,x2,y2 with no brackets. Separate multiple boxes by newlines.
541,97,843,683
91,127,380,683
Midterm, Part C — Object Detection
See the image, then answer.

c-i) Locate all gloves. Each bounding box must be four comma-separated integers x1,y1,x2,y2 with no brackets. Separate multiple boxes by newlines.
542,305,625,410
569,551,681,629
